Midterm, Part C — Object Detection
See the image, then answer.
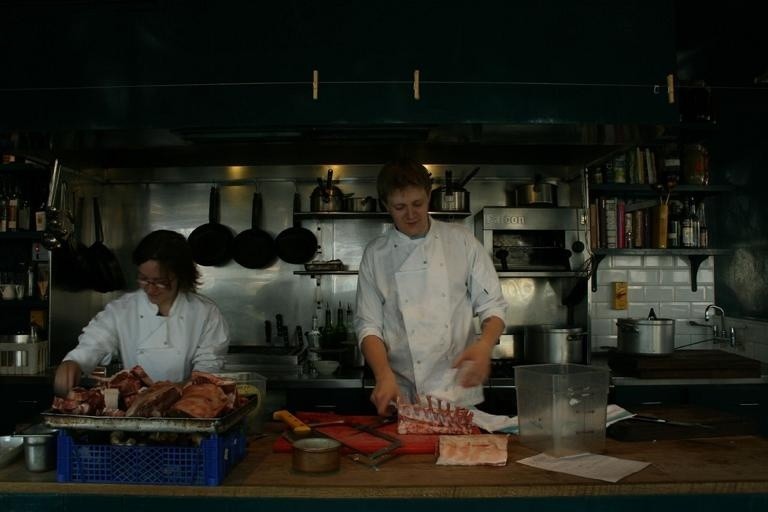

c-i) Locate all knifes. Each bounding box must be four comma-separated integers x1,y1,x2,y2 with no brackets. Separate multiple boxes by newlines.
272,410,320,439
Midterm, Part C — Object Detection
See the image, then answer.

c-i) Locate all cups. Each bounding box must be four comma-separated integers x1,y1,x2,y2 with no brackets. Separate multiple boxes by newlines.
23,435,56,472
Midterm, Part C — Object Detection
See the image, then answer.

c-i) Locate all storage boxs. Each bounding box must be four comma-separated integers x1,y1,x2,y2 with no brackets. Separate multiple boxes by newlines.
511,362,612,460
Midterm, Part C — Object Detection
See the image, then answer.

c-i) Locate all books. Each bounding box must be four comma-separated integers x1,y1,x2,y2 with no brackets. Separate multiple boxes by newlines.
594,145,657,184
591,194,652,252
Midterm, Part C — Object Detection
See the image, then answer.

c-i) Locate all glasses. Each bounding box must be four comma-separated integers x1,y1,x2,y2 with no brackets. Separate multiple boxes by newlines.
134,273,178,289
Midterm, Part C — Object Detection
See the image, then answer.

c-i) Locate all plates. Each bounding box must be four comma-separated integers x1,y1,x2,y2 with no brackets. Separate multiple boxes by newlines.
0,435,25,467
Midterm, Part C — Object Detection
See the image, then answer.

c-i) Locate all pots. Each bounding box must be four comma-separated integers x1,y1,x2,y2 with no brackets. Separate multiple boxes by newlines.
612,306,677,356
510,169,559,206
522,322,586,364
308,164,480,213
185,185,318,269
51,194,128,295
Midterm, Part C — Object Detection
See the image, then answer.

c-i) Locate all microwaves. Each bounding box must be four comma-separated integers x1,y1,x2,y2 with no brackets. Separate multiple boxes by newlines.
473,206,591,280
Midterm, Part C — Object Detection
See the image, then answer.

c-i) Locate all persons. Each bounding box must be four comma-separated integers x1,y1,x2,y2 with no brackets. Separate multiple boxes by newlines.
352,155,509,419
54,229,233,396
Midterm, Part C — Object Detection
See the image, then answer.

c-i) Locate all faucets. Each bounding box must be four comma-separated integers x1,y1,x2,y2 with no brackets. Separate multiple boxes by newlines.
704,303,728,338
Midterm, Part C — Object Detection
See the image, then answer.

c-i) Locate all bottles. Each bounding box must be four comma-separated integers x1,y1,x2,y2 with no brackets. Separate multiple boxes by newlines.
3,286,15,301
308,299,355,348
25,269,34,297
682,142,710,187
626,232,633,249
0,185,46,233
309,352,318,379
591,157,627,185
29,323,41,343
667,197,708,249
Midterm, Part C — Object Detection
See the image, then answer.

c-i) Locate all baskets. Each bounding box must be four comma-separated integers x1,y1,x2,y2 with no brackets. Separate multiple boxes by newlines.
57,425,245,486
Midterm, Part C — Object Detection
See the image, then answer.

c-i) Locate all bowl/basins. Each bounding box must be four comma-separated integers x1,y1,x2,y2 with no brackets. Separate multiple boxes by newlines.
315,360,339,375
608,416,670,441
292,437,342,473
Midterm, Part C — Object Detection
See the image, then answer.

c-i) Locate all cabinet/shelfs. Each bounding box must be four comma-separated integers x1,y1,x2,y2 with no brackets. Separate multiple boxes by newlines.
291,207,471,276
585,174,736,292
0,145,75,389
